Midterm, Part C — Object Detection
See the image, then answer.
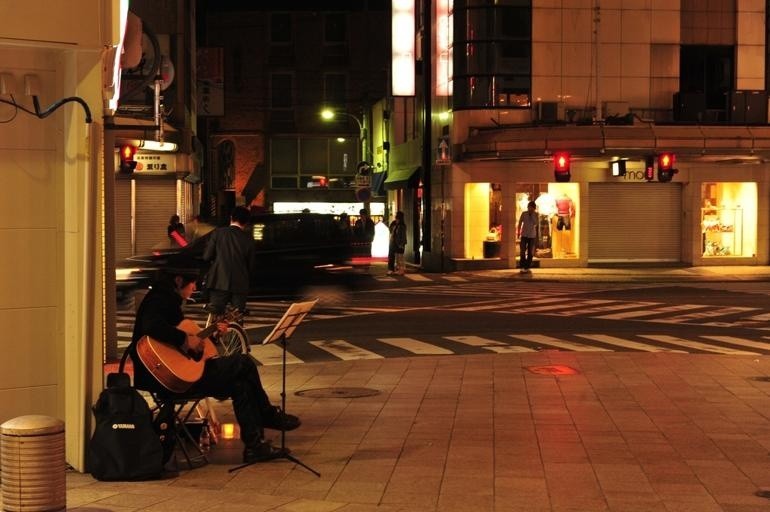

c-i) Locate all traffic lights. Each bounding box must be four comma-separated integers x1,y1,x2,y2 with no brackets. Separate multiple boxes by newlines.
659,153,674,181
118,145,138,175
646,154,654,180
554,152,570,181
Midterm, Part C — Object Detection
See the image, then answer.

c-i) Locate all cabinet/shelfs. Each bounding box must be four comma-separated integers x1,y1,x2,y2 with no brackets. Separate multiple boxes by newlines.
701,207,743,257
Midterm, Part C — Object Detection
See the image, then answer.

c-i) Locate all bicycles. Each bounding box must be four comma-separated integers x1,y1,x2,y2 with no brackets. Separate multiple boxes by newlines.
199,303,250,358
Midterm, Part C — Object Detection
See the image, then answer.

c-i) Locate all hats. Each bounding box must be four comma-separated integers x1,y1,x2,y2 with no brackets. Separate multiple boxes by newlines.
161,254,204,276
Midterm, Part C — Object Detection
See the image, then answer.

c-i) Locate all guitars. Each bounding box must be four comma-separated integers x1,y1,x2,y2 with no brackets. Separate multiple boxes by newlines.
136,304,250,393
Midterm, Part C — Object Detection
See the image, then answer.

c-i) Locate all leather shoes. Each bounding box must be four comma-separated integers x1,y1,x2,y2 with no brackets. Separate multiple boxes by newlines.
264,405,300,431
243,446,291,463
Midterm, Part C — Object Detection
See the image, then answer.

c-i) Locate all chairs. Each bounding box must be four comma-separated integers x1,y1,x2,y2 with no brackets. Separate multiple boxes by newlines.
127,343,210,469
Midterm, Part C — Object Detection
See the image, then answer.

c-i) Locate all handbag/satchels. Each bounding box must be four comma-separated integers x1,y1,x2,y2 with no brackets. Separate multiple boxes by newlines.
90,373,163,481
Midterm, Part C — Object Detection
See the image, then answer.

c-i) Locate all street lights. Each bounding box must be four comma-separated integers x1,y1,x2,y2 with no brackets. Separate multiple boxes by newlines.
318,110,371,221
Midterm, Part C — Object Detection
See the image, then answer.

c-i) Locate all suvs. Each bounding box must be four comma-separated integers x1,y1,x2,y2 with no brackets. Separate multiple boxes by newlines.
114,203,346,310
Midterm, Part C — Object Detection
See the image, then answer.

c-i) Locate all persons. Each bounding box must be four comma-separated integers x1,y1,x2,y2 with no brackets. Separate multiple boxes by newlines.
555,193,575,230
131,266,301,462
353,209,375,275
385,211,406,275
517,201,539,273
191,215,200,242
168,214,185,249
202,206,256,345
